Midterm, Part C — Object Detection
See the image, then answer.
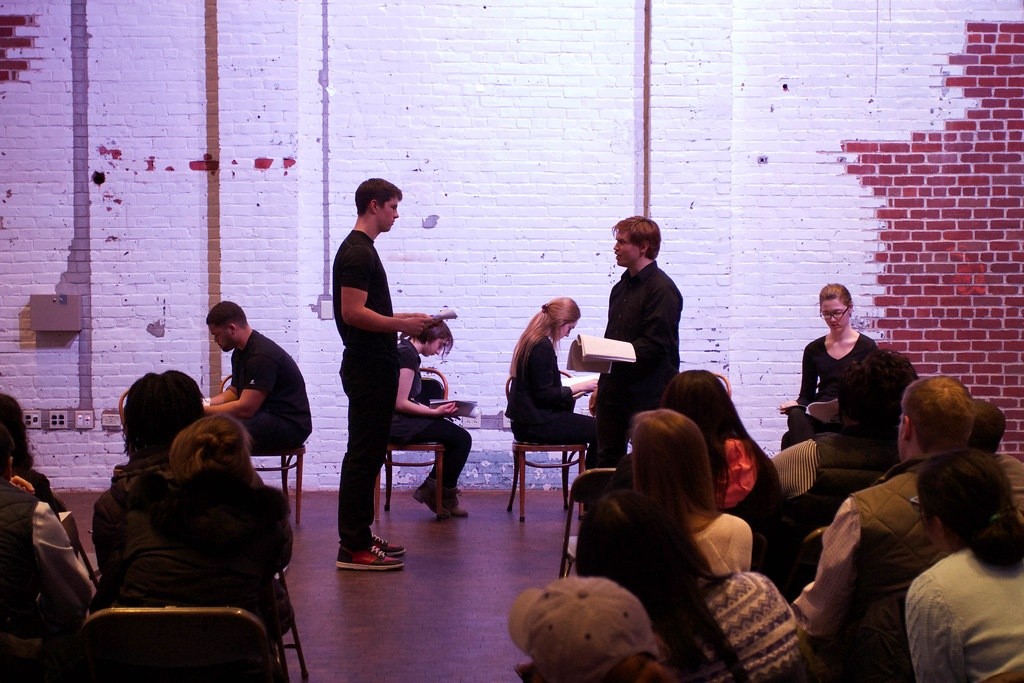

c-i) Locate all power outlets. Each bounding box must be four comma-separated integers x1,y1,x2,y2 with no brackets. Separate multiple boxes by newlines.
503,409,511,428
102,414,122,426
463,410,482,427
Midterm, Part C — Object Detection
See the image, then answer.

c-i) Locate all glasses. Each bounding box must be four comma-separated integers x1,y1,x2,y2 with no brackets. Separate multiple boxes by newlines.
820,304,849,319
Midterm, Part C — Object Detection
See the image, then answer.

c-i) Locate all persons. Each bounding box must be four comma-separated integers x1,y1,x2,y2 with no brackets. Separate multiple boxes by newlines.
120,413,294,645
631,409,754,576
332,178,436,569
969,399,1024,521
776,349,918,503
504,297,596,469
392,315,472,519
661,369,779,515
576,491,808,683
88,372,203,612
903,449,1024,683
780,284,877,451
0,394,90,683
202,301,312,449
582,215,683,469
793,376,977,683
508,576,679,683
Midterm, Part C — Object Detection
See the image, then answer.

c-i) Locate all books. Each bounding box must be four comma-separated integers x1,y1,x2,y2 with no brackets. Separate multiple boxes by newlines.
428,399,476,419
785,398,839,425
562,373,600,398
566,334,636,373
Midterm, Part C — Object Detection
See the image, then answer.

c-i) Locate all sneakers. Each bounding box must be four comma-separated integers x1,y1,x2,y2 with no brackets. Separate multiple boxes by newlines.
443,485,468,518
413,476,450,517
370,533,406,555
336,544,404,570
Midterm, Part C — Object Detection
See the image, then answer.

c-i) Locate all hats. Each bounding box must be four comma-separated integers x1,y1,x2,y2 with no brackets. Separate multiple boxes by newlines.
508,576,659,683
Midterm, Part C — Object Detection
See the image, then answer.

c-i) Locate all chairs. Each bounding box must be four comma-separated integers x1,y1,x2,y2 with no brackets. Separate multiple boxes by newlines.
374,368,448,522
505,370,588,521
559,467,618,579
220,375,304,524
77,607,307,683
